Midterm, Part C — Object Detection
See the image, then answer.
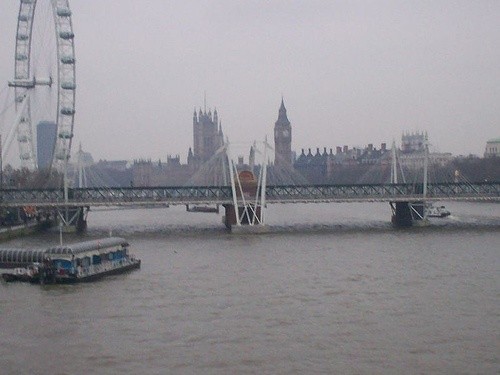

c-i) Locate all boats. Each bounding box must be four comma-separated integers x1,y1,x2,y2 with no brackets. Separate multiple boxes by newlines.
426,206,450,217
186,203,219,213
44,236,141,283
